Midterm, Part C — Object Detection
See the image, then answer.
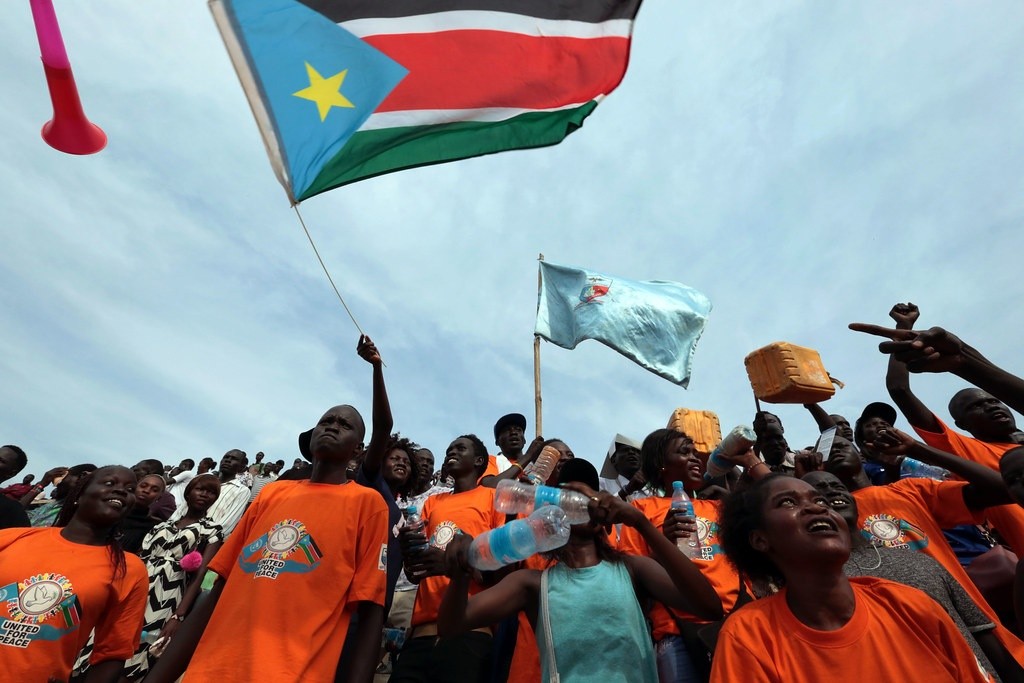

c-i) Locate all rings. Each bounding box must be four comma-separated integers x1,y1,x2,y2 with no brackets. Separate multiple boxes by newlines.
591,496,599,501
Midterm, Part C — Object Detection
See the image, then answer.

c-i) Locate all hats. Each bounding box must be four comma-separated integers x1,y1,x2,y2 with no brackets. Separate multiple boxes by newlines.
494,413,526,438
854,402,897,448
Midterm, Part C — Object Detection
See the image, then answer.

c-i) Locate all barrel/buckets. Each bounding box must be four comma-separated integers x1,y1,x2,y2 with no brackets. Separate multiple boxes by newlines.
666,407,721,454
745,342,836,404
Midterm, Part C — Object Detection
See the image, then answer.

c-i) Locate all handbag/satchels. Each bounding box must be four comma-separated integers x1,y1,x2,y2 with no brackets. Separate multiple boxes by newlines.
962,545,1019,599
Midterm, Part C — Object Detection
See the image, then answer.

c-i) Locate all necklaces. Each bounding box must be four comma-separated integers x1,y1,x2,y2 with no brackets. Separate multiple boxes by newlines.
848,542,882,571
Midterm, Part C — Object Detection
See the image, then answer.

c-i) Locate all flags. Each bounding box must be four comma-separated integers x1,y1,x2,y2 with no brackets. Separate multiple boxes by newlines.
534,260,713,389
223,0,643,201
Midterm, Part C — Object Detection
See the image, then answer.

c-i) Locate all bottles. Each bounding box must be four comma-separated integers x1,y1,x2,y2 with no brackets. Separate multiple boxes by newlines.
895,455,950,482
672,481,702,558
407,506,431,575
493,479,591,525
523,445,561,486
703,424,757,481
469,505,567,570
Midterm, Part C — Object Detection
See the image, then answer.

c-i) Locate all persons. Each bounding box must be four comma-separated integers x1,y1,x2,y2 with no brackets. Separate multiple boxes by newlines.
0,304,1024,683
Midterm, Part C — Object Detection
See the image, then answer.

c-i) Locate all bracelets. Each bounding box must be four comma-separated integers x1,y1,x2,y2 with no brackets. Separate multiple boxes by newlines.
747,461,767,473
512,462,523,471
164,471,168,474
33,486,42,492
618,489,627,498
170,613,185,622
